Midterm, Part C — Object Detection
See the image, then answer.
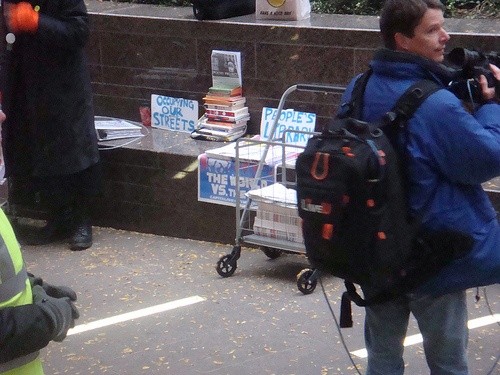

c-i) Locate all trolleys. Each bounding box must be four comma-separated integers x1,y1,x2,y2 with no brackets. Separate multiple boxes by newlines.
217,84,346,295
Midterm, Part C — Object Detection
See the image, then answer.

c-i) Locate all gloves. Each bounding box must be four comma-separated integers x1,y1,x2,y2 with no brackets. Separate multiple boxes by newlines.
28,273,79,342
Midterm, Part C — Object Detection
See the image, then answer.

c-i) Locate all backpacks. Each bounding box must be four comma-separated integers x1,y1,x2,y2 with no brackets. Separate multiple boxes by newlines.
295,71,444,328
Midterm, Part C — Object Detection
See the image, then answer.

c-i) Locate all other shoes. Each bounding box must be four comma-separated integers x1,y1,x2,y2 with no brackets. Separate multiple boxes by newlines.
69,212,91,249
25,216,68,245
